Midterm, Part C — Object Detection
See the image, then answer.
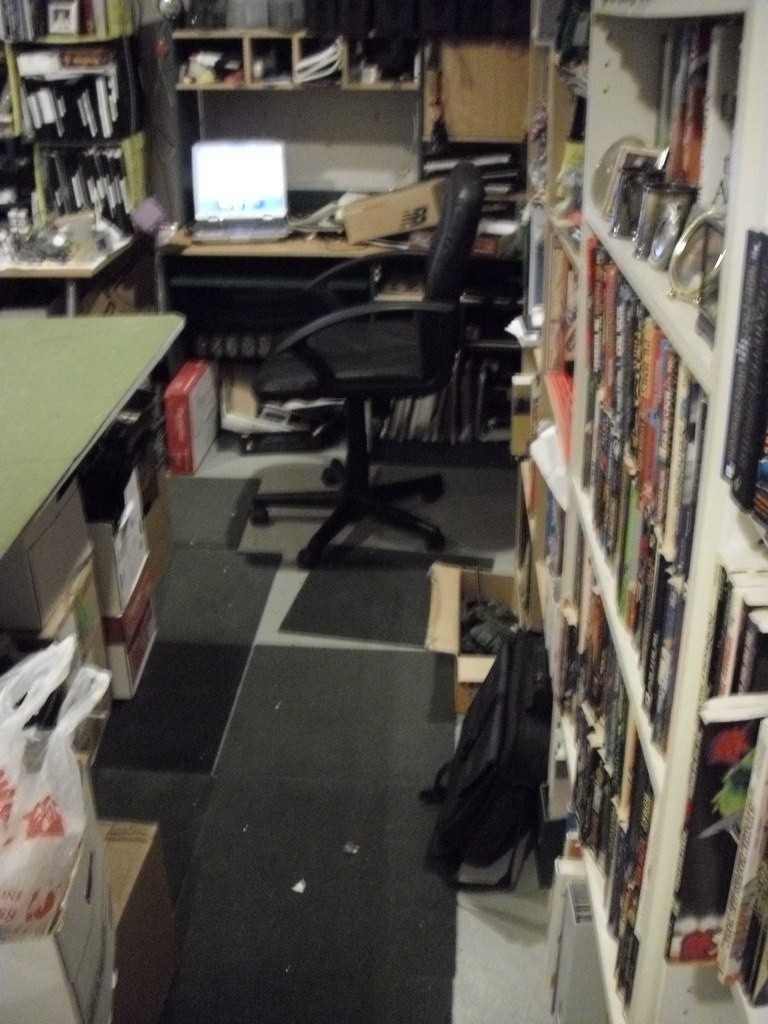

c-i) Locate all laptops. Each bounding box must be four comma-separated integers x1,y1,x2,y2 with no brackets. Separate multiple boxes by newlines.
189,139,289,243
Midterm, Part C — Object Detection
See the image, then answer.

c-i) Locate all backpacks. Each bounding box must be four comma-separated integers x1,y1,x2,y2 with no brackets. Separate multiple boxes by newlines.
418,627,552,892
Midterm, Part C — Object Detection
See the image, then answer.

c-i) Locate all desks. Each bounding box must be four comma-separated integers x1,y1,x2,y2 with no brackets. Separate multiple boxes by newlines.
154,239,392,379
0,312,184,559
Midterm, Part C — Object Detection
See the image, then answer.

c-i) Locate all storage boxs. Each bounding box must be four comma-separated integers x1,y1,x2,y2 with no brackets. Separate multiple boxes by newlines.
88,362,215,700
426,561,518,716
0,757,177,1024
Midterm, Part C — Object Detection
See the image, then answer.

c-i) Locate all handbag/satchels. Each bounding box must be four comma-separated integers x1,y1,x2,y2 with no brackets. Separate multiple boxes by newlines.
0,633,113,936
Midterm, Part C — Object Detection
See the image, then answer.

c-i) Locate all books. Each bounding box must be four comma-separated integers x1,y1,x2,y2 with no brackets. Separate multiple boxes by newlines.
0,0,768,1024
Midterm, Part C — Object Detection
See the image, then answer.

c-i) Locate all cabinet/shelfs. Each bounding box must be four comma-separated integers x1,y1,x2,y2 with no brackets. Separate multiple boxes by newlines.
170,26,423,92
516,3,768,1023
367,252,530,463
0,0,150,314
422,2,529,143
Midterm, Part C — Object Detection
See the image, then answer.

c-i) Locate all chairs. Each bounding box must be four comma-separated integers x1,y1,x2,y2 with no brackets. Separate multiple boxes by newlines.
250,163,487,571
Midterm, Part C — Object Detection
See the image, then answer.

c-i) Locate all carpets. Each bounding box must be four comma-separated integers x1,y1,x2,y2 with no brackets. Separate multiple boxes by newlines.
90,478,493,1024
